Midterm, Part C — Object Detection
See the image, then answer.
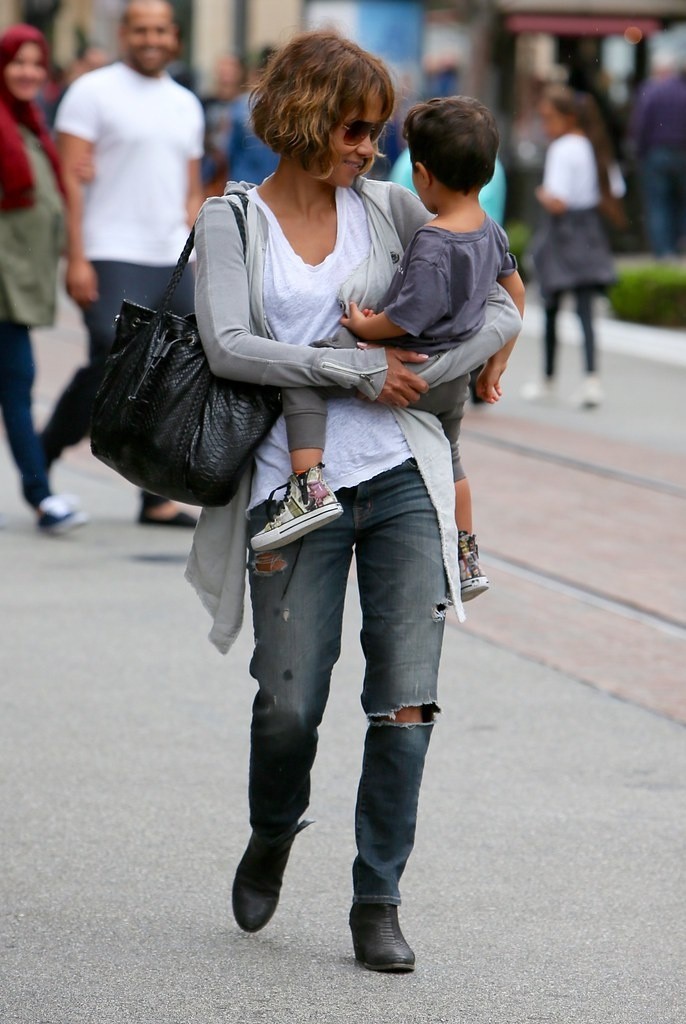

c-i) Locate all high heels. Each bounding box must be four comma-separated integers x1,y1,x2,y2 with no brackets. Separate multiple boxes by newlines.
349,901,416,971
231,818,315,933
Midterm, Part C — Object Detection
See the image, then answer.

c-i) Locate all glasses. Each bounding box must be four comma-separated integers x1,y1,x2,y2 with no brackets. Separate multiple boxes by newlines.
342,120,384,145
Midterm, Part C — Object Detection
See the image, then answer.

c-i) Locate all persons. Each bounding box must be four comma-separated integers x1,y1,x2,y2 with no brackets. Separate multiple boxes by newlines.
251,95,525,614
0,24,93,534
626,50,686,256
526,85,625,407
184,30,524,969
390,147,506,228
199,45,281,199
39,0,206,529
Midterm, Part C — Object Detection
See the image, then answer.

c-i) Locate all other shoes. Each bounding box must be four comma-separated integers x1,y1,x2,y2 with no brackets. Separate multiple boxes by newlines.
526,382,564,398
41,500,88,533
581,383,601,403
139,510,199,527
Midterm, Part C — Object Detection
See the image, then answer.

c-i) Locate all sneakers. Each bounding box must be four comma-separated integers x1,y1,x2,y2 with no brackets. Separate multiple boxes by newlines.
250,462,344,552
448,531,488,606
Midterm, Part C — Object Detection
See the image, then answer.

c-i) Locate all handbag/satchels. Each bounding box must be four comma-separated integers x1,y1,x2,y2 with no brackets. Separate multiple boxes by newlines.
88,193,284,509
